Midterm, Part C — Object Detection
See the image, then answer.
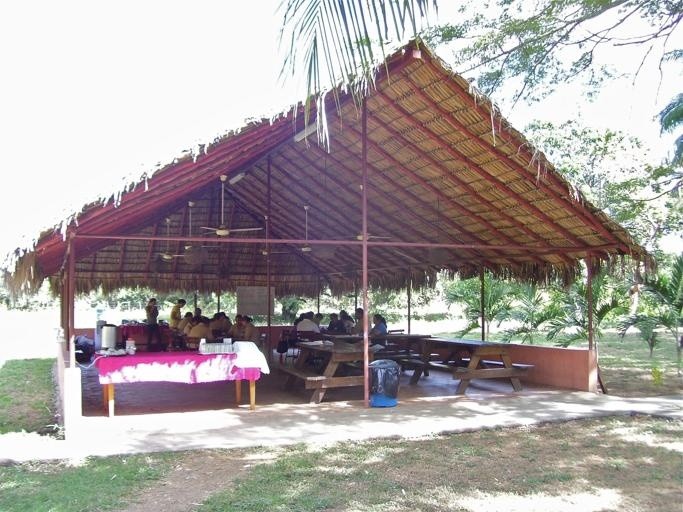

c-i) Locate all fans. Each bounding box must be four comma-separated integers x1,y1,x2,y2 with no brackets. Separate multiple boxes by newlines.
150,174,391,261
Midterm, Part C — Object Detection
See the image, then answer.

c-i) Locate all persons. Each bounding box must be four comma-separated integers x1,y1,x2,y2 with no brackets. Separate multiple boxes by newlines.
169,299,187,350
144,298,162,351
180,308,258,348
293,304,387,366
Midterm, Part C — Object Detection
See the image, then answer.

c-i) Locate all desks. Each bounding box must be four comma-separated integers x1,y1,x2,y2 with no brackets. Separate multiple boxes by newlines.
277,340,375,405
93,350,260,417
118,323,173,351
278,324,430,376
402,338,531,394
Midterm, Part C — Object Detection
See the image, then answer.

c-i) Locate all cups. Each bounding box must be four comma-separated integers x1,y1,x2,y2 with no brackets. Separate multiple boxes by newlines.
199,342,233,352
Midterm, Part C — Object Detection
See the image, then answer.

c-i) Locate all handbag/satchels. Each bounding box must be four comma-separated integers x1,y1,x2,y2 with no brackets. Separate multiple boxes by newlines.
277,340,288,353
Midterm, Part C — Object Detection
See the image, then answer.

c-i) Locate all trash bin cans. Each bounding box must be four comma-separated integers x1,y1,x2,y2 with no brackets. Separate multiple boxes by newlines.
368,359,400,406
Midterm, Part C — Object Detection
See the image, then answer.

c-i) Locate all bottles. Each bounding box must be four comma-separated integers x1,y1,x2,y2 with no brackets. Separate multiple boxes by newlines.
125,337,135,355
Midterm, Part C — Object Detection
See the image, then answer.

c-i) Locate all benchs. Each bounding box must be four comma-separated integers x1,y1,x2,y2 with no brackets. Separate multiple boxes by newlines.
400,357,534,379
278,361,371,391
